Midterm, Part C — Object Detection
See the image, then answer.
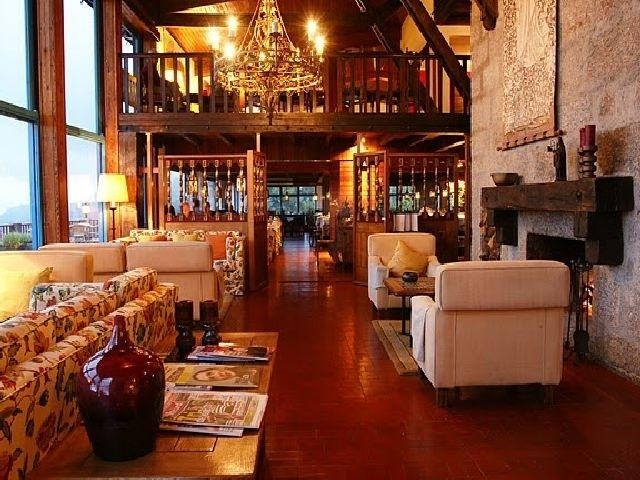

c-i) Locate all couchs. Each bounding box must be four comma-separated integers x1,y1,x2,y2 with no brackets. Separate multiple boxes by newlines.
0,282,182,479
113,229,245,296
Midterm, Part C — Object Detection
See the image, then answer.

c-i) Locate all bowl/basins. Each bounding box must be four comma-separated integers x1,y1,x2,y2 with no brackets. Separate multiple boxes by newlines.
489,172,519,186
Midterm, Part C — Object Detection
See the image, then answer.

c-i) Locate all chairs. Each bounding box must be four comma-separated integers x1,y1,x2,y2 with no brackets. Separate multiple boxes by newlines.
140,74,173,112
1,250,94,282
126,241,225,322
410,259,572,408
38,242,126,282
368,232,441,310
266,210,354,273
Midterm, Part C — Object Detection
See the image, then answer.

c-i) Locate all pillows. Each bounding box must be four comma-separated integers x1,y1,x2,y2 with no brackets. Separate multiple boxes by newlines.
43,291,118,342
138,235,167,243
205,235,227,260
225,236,235,260
171,233,198,241
1,311,57,379
102,267,159,307
388,240,428,278
0,255,53,322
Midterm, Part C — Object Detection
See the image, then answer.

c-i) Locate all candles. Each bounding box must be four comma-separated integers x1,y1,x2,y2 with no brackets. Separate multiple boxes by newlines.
200,299,219,323
586,123,596,145
175,299,193,321
580,127,586,147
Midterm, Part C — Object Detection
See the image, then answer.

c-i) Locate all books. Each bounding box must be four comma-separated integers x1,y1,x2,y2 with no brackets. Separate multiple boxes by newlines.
151,341,273,439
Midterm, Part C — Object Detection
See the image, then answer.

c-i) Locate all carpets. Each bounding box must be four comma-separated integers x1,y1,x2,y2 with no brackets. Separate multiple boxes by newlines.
371,319,422,375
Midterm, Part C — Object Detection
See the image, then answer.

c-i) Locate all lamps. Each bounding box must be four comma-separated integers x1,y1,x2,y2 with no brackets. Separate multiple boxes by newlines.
96,173,129,242
217,0,319,125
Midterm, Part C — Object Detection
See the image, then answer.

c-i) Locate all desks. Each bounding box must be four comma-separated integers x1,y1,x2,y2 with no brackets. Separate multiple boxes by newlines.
26,332,278,479
383,276,435,334
